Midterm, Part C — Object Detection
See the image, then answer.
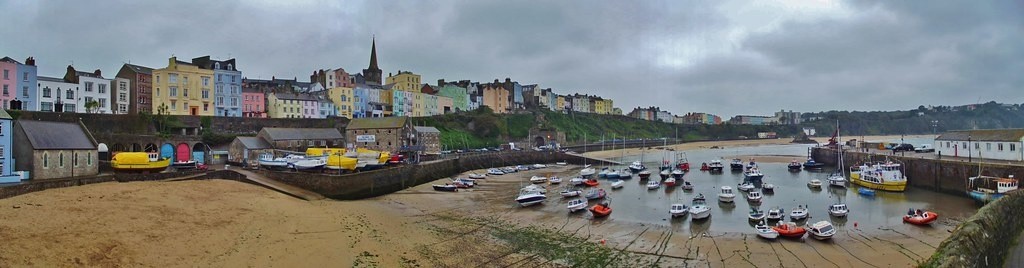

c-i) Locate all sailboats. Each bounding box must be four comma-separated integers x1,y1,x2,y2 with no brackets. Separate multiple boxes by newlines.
827,119,847,188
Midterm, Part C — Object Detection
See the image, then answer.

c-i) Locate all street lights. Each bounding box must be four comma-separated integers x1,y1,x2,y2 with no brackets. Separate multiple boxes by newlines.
932,120,938,140
969,133,972,162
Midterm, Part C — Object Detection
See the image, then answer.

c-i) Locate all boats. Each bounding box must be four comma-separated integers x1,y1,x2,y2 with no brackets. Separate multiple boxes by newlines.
788,163,801,170
287,147,407,171
903,211,939,225
106,151,172,174
967,174,1018,202
827,205,851,219
803,158,824,169
257,154,289,167
669,194,712,221
551,176,561,184
748,205,836,242
515,185,547,206
170,161,209,169
571,130,660,192
730,158,774,202
700,160,725,174
660,129,693,189
469,173,489,179
433,179,478,191
850,156,909,196
808,179,821,189
717,186,737,204
489,164,547,175
563,189,612,215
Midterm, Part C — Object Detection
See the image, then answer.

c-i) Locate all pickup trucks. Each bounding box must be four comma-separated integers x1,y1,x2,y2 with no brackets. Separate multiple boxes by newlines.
915,143,935,152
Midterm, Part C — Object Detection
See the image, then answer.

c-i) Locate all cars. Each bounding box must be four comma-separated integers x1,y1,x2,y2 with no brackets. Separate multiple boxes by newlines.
891,143,914,152
